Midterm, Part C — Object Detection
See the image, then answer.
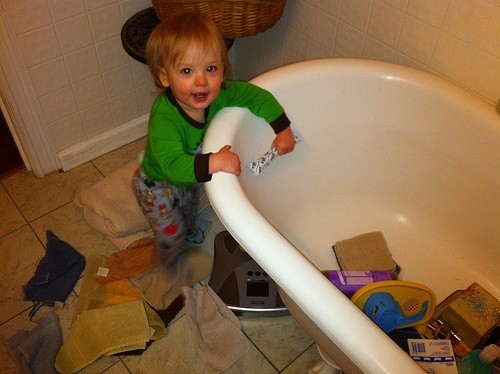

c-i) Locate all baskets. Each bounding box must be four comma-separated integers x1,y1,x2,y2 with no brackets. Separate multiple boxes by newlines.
152,0,286,38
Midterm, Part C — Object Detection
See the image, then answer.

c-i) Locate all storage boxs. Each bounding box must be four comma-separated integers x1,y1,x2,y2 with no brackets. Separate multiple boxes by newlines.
440,282,500,349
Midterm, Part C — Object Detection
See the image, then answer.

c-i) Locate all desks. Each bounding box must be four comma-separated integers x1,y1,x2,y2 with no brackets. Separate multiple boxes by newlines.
121,7,235,64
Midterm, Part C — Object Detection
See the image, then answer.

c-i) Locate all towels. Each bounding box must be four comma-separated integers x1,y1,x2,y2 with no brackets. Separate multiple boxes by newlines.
4,310,63,374
53,300,156,374
73,157,157,251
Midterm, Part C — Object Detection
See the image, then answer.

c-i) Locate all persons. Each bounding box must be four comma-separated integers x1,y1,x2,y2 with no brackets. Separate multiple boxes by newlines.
132,14,296,289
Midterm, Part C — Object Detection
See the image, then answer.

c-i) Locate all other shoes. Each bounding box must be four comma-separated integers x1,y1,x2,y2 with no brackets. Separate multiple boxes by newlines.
188,227,205,245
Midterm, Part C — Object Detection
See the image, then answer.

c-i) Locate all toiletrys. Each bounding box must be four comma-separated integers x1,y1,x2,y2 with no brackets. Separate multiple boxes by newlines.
325,269,395,292
458,343,500,374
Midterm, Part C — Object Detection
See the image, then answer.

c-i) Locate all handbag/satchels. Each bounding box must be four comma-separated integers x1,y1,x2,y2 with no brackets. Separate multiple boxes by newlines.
24,230,86,308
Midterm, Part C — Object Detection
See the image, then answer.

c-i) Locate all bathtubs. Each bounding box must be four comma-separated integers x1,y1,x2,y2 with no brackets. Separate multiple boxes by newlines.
197,55,500,374
